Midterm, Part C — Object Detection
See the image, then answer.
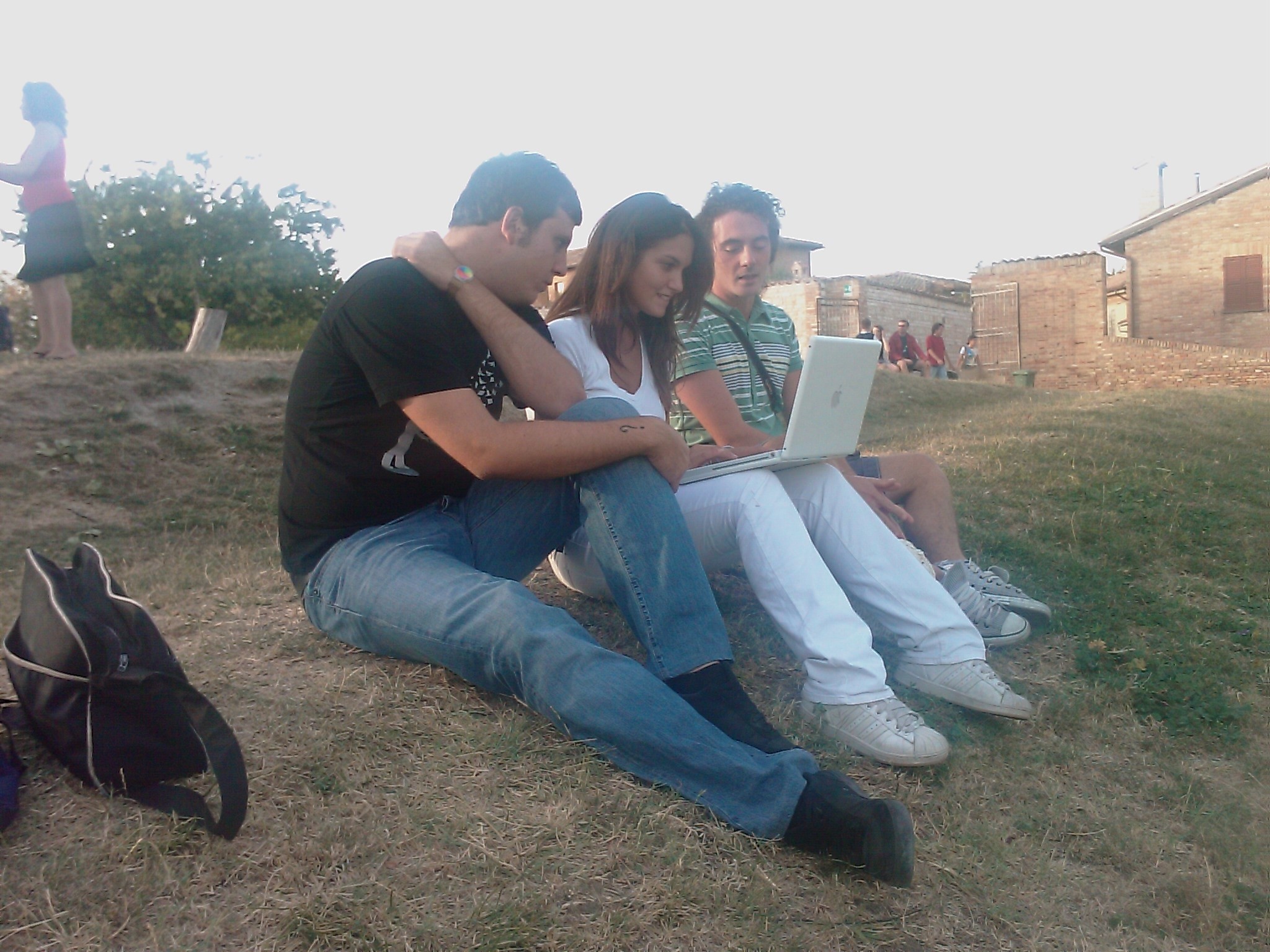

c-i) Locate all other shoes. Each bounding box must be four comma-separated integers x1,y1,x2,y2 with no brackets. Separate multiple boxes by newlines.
47,343,79,361
27,343,52,357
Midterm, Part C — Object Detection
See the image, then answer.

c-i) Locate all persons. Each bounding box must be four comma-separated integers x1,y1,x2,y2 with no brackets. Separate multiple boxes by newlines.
665,181,1051,649
523,191,1035,764
855,318,878,340
269,151,918,885
959,335,983,364
887,320,930,379
0,79,97,361
871,325,900,373
925,323,951,380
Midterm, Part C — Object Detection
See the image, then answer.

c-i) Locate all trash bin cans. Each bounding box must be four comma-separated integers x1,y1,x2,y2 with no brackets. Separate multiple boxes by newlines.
1010,370,1037,387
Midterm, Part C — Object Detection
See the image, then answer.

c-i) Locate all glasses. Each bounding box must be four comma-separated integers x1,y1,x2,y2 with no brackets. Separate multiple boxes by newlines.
897,324,907,327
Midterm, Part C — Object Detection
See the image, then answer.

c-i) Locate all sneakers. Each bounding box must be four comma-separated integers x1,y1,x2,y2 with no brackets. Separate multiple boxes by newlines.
793,764,915,887
894,653,1034,725
796,685,950,769
950,557,1053,627
940,562,1033,648
666,660,801,758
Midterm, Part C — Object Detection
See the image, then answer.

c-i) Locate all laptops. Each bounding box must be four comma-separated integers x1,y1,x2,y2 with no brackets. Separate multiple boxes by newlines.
679,335,882,486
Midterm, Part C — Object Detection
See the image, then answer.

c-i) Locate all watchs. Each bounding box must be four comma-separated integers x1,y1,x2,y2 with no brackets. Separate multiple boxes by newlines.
447,265,476,294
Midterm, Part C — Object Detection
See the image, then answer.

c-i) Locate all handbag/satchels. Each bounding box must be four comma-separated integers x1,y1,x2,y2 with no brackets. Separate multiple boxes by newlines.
1,540,250,843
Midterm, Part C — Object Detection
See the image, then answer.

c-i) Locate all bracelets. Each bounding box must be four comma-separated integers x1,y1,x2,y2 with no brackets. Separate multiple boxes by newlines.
722,446,735,449
